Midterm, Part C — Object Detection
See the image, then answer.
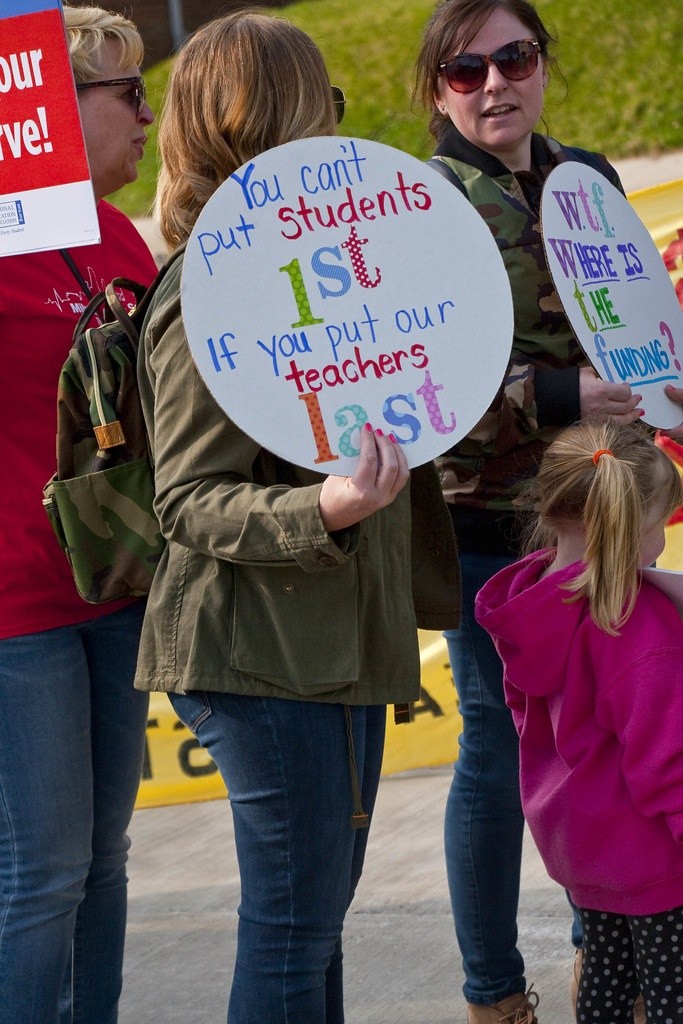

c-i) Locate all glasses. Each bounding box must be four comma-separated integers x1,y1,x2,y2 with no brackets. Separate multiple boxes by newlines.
331,87,347,125
437,39,542,95
76,76,146,114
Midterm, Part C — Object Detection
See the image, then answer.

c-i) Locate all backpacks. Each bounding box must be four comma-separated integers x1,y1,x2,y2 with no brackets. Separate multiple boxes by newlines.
43,238,190,605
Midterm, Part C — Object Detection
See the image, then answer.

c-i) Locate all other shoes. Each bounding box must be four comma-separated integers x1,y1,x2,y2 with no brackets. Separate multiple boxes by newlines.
467,983,539,1024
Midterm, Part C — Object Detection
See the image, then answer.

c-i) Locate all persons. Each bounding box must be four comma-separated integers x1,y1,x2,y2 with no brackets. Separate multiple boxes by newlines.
136,8,462,1024
414,0,683,1024
474,414,683,1024
0,5,160,1024
